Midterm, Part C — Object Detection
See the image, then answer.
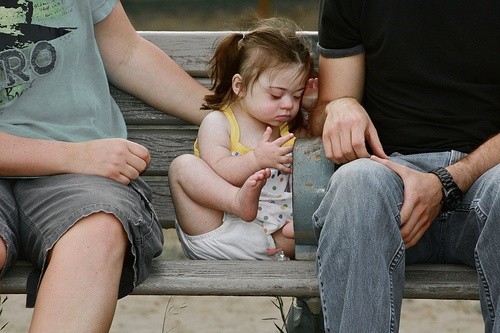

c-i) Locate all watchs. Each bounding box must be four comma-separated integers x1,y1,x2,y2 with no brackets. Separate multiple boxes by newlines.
428,167,464,212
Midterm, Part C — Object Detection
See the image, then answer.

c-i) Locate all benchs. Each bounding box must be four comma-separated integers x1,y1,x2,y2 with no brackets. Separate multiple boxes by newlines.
0,31,480,333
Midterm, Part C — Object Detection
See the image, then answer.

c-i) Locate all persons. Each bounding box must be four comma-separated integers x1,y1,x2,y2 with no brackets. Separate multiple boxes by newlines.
308,1,500,333
0,0,222,333
168,16,318,261
